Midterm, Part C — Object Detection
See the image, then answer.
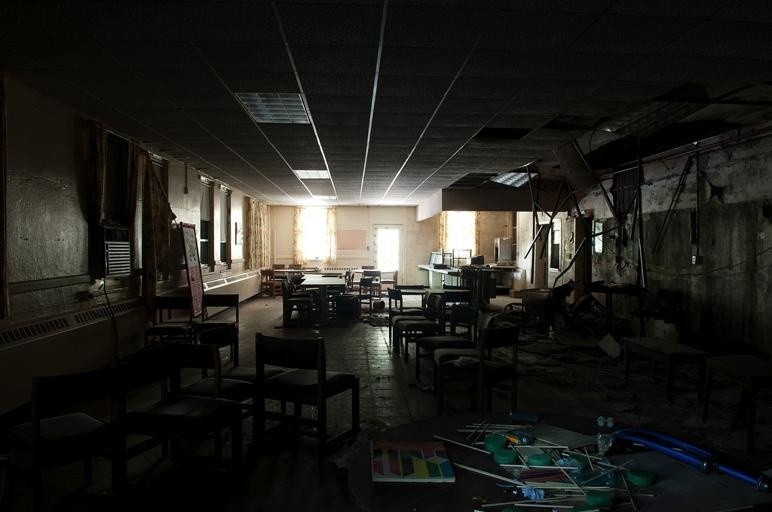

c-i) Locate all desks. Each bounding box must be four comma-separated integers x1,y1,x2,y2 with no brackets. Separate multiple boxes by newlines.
622,337,711,405
698,355,772,454
349,414,772,512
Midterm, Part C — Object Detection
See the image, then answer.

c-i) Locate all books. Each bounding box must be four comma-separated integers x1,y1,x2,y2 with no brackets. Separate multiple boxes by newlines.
369,438,457,484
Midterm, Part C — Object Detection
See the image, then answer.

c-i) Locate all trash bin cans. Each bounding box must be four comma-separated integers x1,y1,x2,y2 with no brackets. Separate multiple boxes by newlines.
336,296,358,322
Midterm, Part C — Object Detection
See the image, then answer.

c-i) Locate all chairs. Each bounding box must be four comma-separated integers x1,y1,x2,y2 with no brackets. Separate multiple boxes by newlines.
385,264,605,415
256,263,399,325
2,293,361,510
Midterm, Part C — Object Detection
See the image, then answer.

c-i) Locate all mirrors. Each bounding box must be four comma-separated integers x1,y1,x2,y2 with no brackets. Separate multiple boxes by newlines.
100,126,132,232
